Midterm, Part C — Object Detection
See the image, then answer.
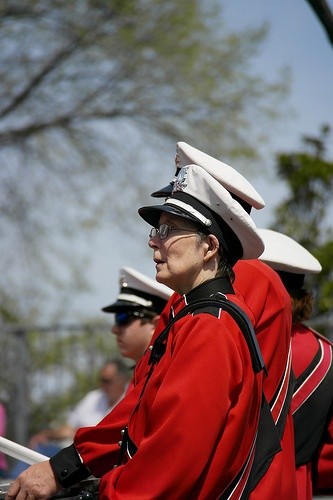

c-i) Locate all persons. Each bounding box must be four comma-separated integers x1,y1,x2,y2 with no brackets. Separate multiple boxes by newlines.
10,141,293,500
0,403,8,483
252,229,332,500
11,359,133,477
110,267,173,361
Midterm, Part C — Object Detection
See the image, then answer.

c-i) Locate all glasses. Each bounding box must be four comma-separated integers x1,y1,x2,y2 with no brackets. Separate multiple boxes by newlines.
115,311,156,326
149,224,207,240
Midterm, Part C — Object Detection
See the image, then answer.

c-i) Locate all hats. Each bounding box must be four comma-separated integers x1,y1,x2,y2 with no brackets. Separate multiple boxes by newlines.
151,141,266,215
102,267,177,317
138,164,264,260
254,229,322,289
